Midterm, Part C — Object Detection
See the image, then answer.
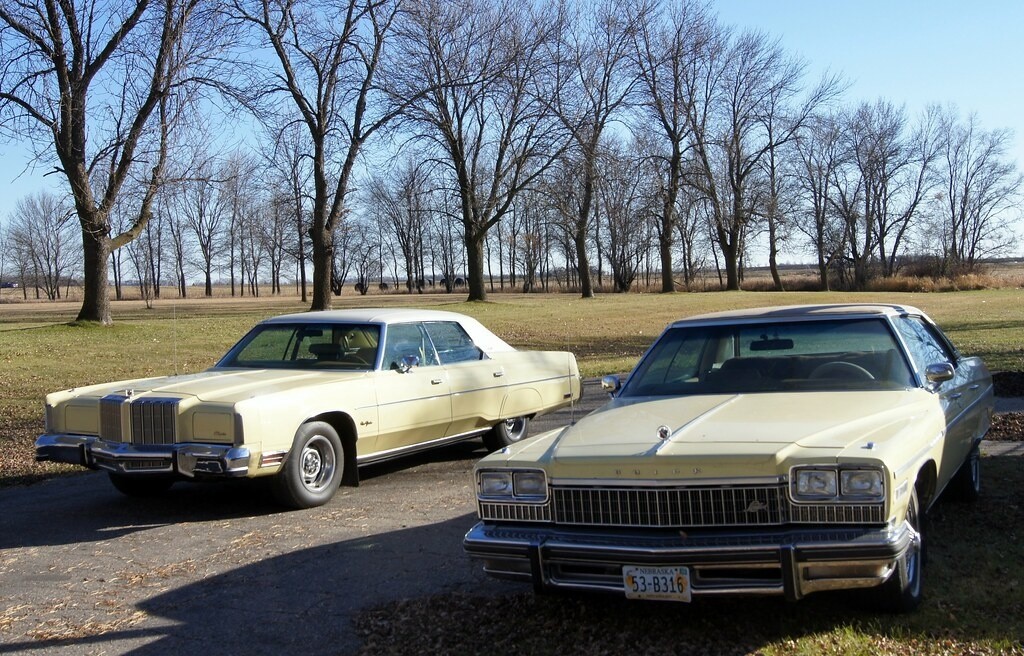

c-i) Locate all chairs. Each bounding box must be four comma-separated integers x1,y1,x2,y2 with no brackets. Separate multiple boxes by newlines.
841,348,900,387
309,342,344,364
387,340,428,371
354,346,380,367
707,354,780,395
783,353,842,387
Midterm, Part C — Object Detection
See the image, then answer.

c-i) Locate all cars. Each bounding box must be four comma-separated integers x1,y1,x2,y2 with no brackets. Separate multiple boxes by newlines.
36,306,582,511
463,302,996,615
